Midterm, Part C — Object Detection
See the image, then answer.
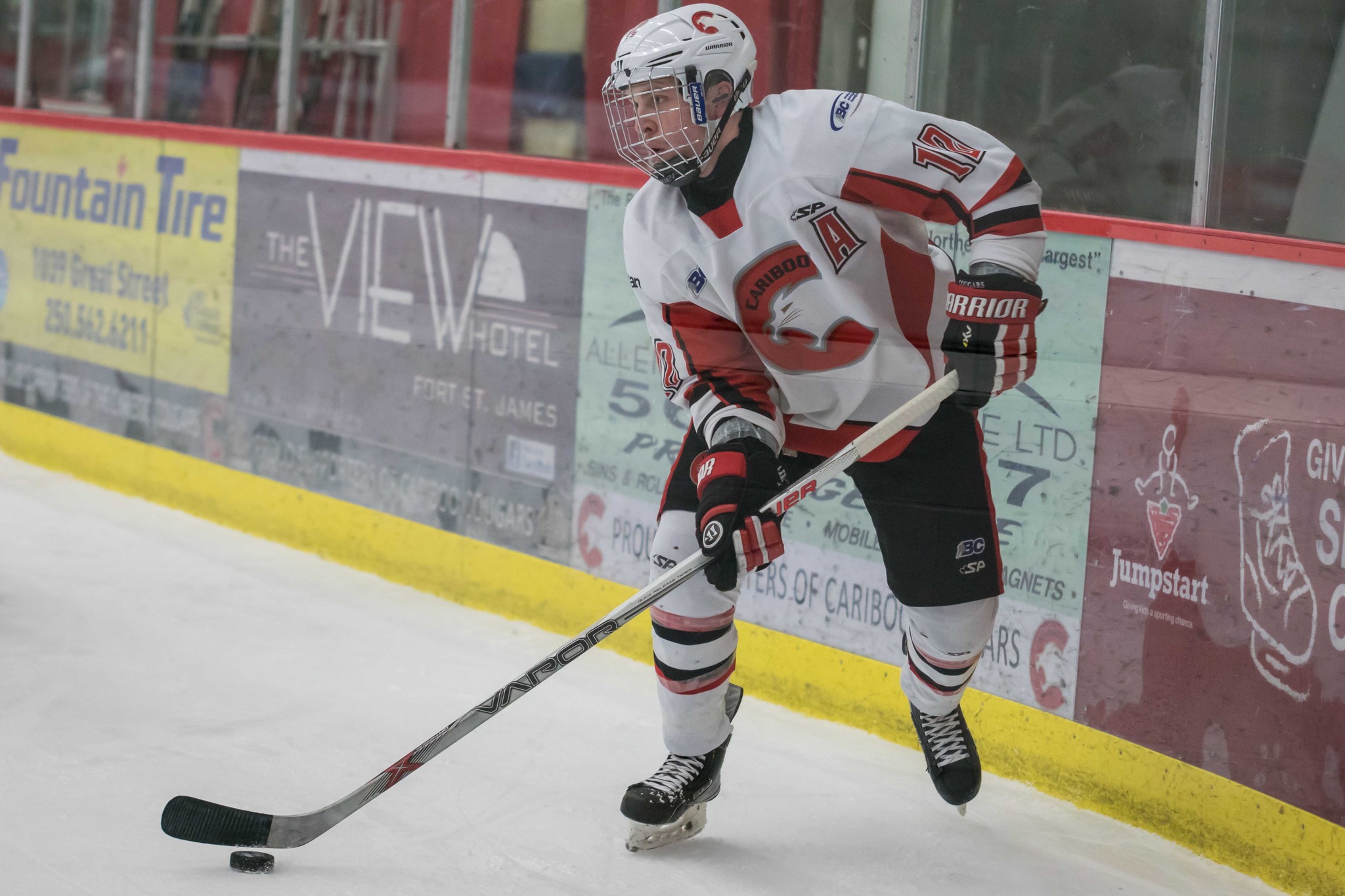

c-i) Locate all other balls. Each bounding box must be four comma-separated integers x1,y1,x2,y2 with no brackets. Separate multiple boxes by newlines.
227,851,276,872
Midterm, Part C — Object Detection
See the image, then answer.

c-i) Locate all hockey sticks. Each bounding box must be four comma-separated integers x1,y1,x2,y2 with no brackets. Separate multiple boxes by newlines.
159,372,959,849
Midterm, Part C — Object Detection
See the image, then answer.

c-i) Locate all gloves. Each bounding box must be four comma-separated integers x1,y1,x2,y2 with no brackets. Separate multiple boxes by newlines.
938,269,1047,411
689,436,784,592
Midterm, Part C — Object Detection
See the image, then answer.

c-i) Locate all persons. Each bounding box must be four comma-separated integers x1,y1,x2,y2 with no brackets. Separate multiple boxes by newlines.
600,2,1045,854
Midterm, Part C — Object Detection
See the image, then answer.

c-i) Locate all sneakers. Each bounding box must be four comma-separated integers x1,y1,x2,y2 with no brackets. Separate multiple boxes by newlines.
909,699,982,815
621,681,742,854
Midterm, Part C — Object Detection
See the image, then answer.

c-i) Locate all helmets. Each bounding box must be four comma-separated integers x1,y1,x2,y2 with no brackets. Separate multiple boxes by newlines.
602,3,756,187
